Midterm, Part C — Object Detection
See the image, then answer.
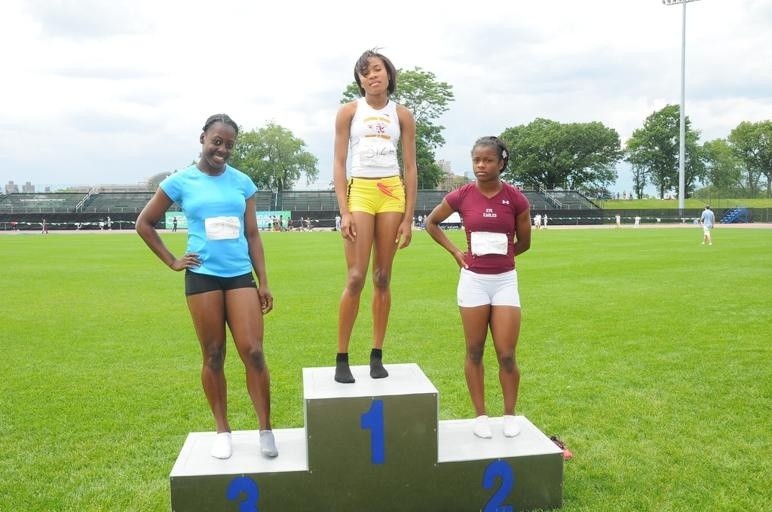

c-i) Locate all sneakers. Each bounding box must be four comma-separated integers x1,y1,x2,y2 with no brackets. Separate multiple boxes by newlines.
552,434,572,459
709,242,712,244
700,241,705,245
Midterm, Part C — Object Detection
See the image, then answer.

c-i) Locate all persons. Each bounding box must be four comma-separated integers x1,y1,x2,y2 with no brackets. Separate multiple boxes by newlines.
699,204,715,246
107,215,114,230
634,214,641,228
132,113,281,463
40,216,49,235
329,48,418,385
172,217,178,232
98,217,105,232
533,212,548,232
614,213,621,228
268,214,320,233
423,134,532,441
334,214,342,233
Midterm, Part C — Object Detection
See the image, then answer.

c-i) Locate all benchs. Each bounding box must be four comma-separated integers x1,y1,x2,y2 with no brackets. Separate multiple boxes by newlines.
0,187,604,214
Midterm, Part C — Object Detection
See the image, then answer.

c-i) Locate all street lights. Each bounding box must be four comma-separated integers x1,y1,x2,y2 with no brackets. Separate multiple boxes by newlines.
662,0,701,217
